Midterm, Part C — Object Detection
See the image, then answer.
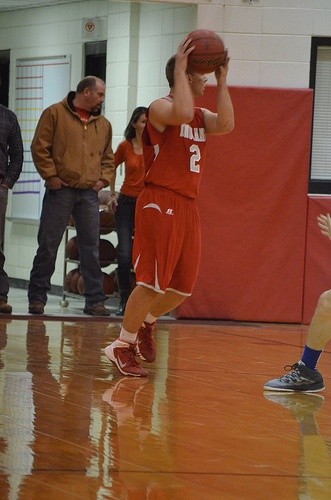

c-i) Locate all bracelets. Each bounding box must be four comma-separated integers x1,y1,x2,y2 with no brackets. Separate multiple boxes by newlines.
109,194,116,197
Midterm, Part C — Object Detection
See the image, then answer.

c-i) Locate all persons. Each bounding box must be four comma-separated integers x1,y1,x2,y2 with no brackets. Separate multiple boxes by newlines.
262,213,331,393
30,75,115,317
0,105,23,312
106,107,151,318
99,33,235,379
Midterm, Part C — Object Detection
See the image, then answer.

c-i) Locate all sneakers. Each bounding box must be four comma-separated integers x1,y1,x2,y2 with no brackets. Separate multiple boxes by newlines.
263,363,325,393
83,300,112,316
103,338,148,378
28,301,44,313
137,317,158,363
0,296,13,314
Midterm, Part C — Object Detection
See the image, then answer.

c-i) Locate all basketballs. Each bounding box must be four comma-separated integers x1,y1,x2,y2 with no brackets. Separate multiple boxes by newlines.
67,236,120,268
64,268,136,300
99,211,115,235
183,29,225,74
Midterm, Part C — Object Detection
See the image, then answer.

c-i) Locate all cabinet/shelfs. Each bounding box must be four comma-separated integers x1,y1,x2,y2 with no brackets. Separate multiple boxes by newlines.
60,226,136,307
